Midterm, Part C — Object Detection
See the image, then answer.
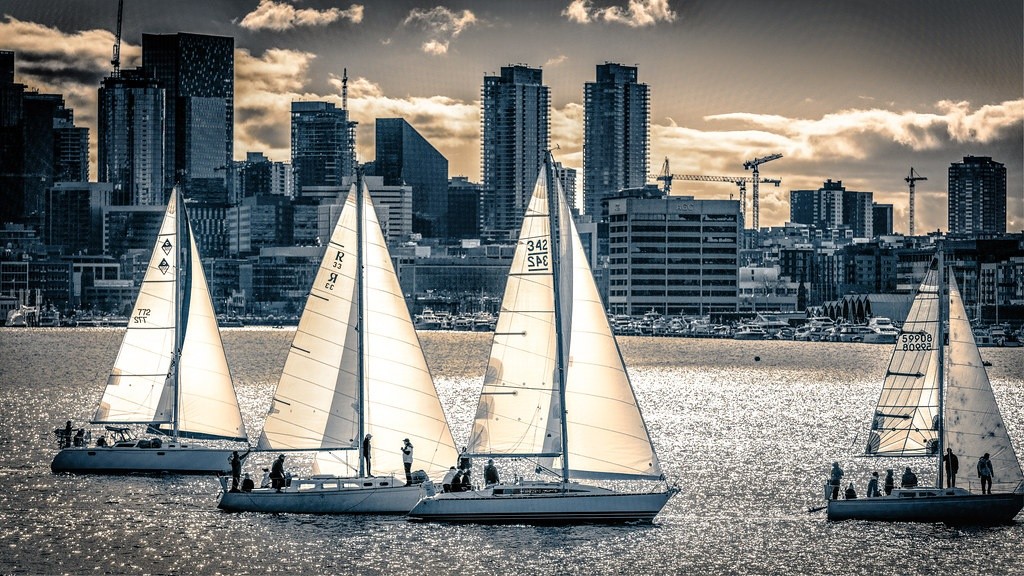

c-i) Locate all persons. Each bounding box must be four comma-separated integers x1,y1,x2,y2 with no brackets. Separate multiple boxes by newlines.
64,421,78,447
74,429,85,446
977,453,994,494
867,472,879,497
242,474,253,491
401,438,413,487
944,448,958,487
885,470,893,494
363,434,373,477
232,449,251,490
901,467,918,487
261,467,270,487
272,454,286,493
830,462,844,499
484,459,499,485
98,435,107,447
845,483,857,499
442,447,472,492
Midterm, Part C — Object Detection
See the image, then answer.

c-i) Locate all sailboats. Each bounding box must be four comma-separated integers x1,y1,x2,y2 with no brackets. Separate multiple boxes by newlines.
825,237,1024,527
49,182,260,474
219,162,461,516
404,146,683,522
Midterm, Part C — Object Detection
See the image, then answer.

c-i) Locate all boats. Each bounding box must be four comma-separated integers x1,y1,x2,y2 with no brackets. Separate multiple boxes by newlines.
606,302,901,345
942,317,1024,347
4,304,130,327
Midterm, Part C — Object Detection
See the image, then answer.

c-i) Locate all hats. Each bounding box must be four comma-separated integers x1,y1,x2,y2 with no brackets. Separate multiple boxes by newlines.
403,438,410,442
366,434,373,437
279,454,286,457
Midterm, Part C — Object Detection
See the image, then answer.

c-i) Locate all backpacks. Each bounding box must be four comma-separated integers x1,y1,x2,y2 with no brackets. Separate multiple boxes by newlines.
979,459,990,476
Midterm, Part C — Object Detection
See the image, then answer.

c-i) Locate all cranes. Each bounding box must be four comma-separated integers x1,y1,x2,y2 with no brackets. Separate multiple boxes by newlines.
904,166,928,236
743,152,784,250
656,155,780,196
